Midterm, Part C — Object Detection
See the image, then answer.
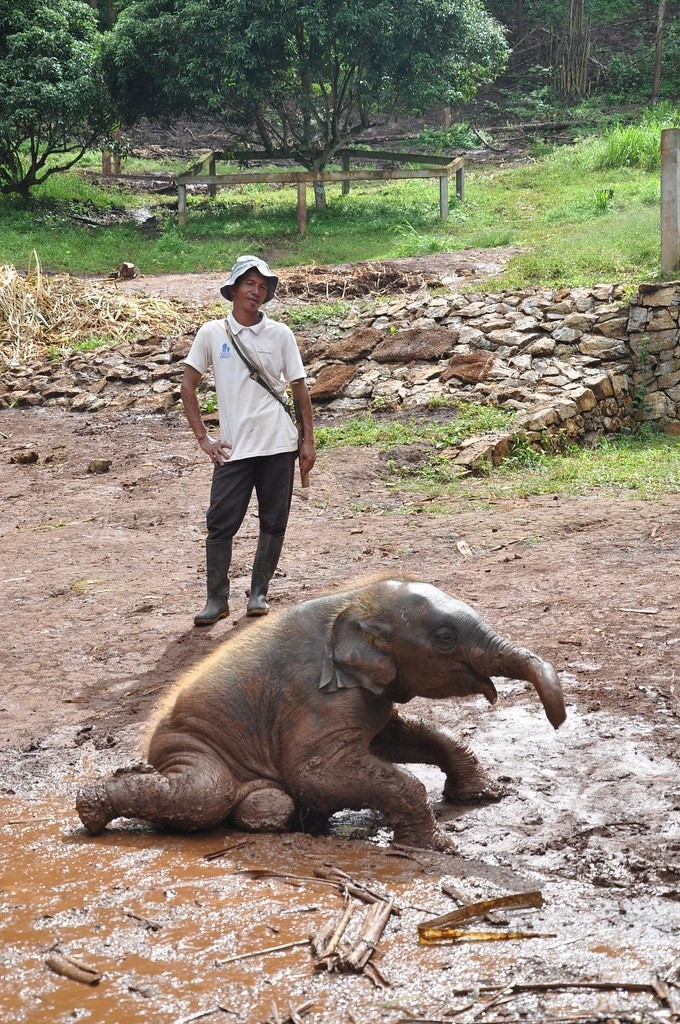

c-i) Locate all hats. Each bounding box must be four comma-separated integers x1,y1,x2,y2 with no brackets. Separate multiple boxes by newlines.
220,255,279,304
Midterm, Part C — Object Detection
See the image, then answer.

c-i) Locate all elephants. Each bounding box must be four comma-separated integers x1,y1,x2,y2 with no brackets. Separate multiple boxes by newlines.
75,572,567,851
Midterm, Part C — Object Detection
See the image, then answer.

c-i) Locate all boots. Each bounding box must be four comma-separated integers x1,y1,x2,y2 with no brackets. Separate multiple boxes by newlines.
193,535,233,625
246,530,286,616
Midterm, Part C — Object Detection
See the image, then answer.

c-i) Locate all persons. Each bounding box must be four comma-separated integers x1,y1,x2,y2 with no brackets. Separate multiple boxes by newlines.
180,254,316,625
